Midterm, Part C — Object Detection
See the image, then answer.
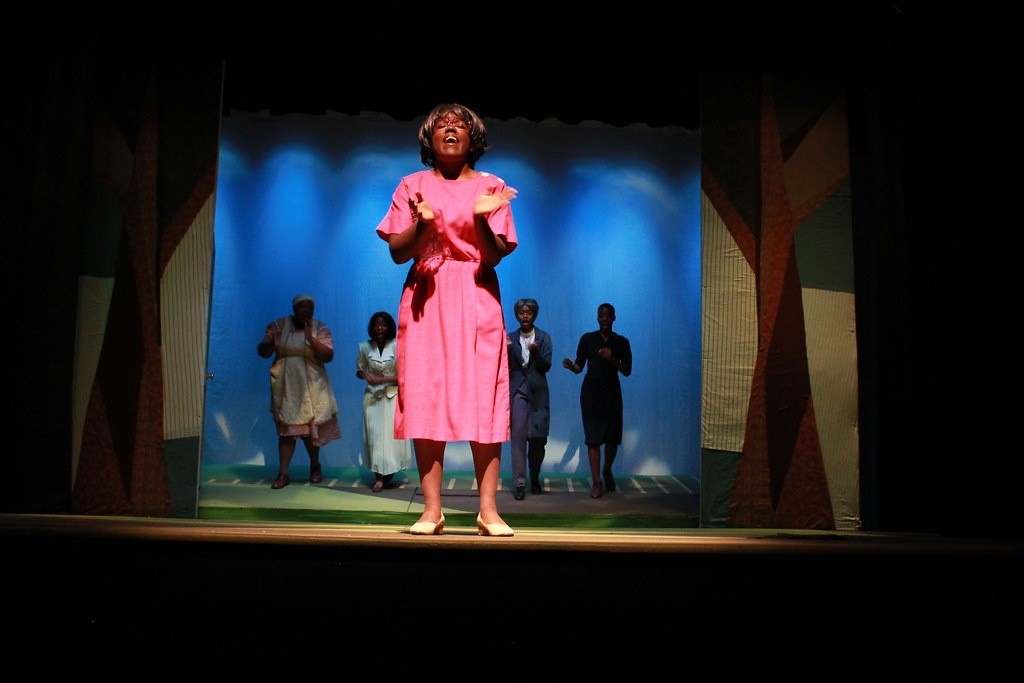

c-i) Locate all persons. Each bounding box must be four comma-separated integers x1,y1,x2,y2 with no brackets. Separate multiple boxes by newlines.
355,311,413,492
562,303,633,499
503,297,553,500
375,104,517,536
256,294,342,490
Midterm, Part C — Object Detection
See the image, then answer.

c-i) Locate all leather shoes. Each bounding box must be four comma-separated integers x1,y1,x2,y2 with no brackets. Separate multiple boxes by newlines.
602,469,616,491
590,479,603,498
531,479,541,494
514,485,525,499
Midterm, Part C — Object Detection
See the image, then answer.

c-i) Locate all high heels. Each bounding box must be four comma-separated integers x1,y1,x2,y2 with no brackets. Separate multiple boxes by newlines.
309,463,323,483
410,510,445,534
272,473,289,488
477,512,514,536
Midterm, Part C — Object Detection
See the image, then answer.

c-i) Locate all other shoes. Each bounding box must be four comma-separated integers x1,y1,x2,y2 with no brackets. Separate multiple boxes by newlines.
373,476,384,491
